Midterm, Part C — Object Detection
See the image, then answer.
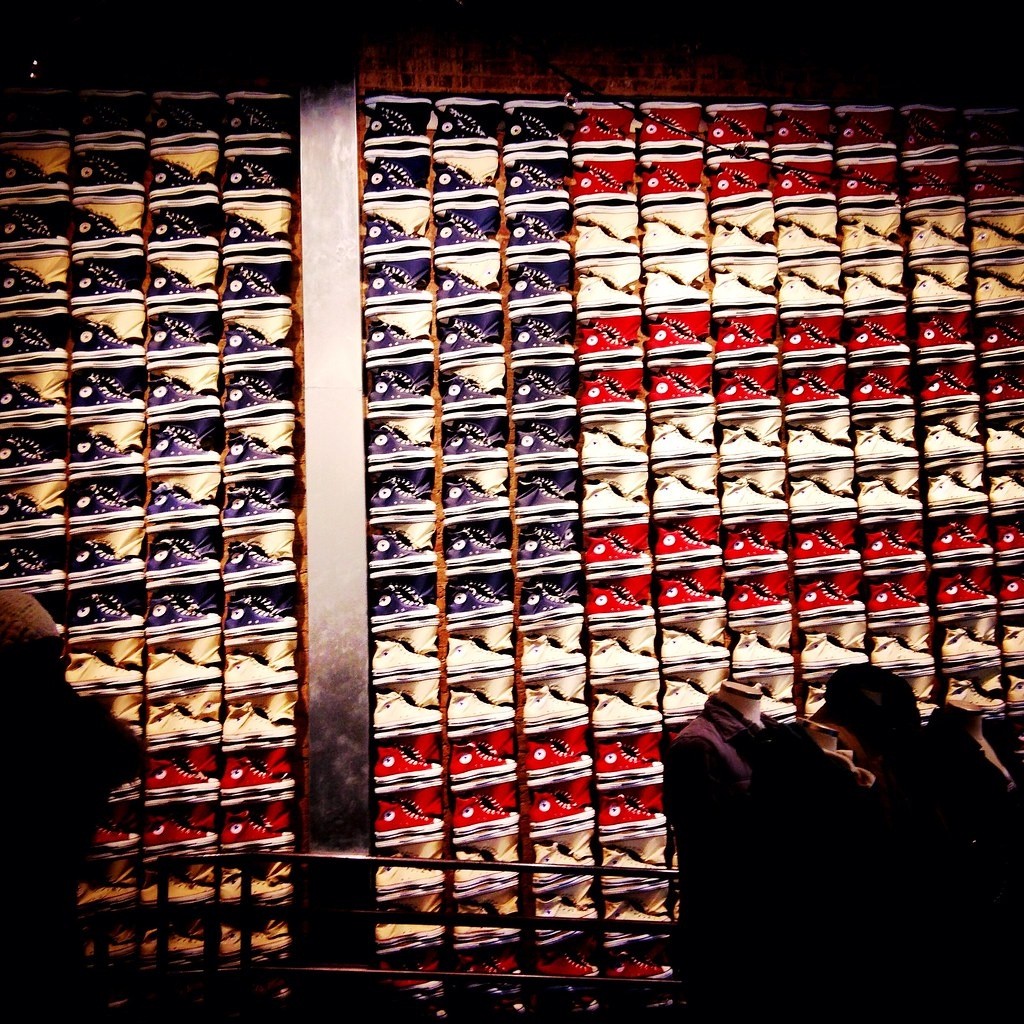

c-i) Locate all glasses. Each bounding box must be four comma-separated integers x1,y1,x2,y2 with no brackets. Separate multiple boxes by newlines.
0,634,69,673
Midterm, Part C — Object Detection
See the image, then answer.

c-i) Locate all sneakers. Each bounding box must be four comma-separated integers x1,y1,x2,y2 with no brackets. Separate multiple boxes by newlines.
0,93,69,634
373,738,674,1018
66,89,299,1016
361,99,1024,739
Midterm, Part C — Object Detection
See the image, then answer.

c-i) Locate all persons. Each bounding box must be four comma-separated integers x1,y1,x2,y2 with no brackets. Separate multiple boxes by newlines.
666,667,1024,1023
1,590,141,1023
